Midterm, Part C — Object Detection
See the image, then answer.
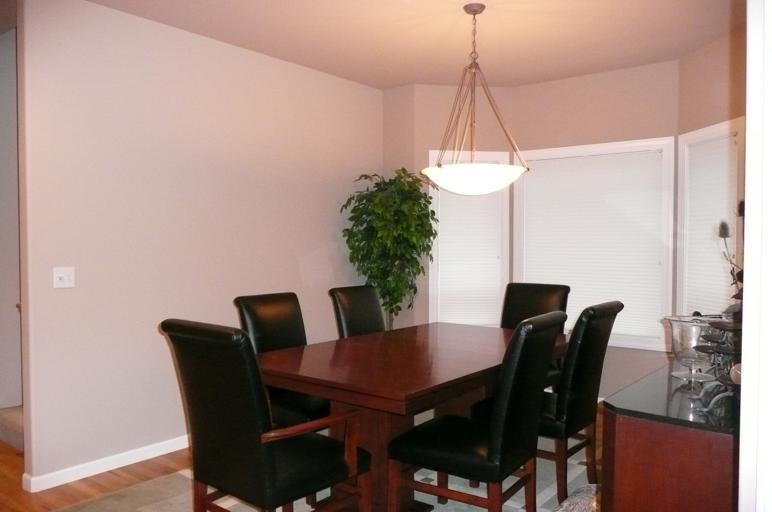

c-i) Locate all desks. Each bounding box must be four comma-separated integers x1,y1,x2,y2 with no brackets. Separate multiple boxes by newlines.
601,354,739,512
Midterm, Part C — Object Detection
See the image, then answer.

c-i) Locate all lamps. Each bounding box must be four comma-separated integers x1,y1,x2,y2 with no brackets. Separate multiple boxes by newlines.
420,3,530,196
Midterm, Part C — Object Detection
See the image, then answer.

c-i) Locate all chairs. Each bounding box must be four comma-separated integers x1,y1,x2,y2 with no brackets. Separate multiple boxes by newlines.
327,284,386,339
500,282,570,333
385,310,567,512
468,300,625,504
232,292,330,512
159,318,372,512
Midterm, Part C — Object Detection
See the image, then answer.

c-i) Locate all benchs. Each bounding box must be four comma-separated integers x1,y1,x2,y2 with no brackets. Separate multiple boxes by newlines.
254,321,572,512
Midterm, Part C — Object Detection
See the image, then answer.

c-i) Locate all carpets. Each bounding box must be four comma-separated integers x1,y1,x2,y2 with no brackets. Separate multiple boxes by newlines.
52,427,600,512
